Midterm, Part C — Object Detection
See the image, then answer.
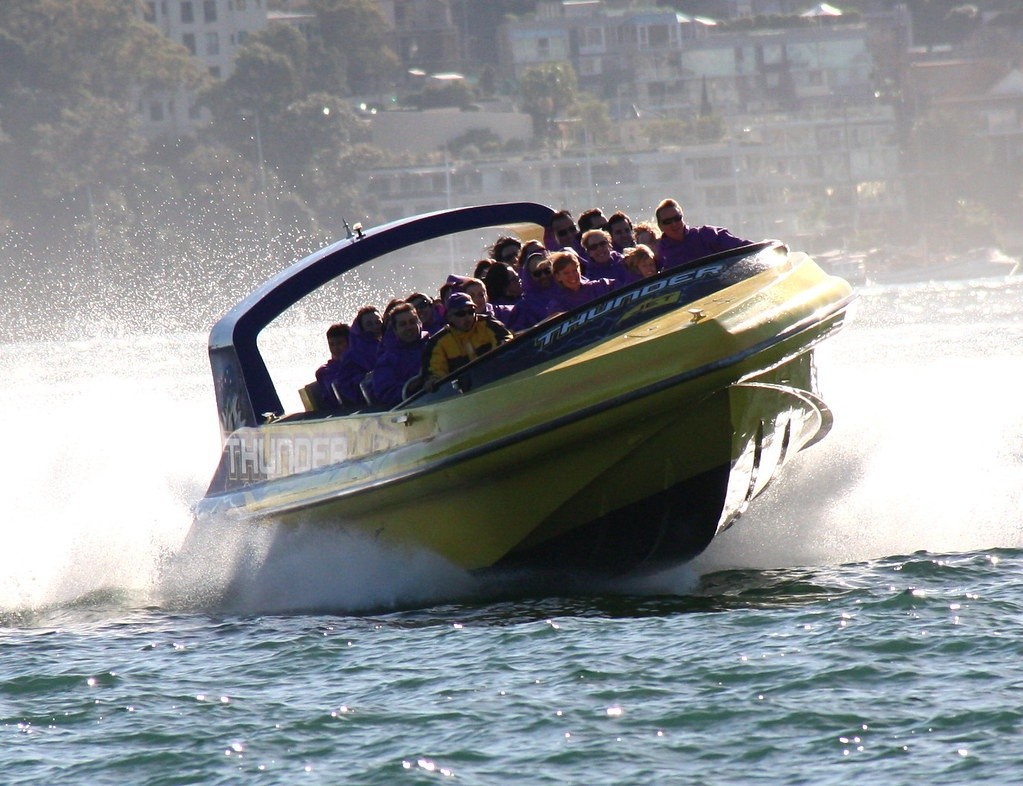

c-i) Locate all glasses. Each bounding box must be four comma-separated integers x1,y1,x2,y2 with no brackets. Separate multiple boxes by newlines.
530,267,551,279
454,308,476,318
530,247,548,255
415,299,429,309
661,215,681,226
499,251,522,263
509,277,520,285
589,240,608,252
556,225,577,237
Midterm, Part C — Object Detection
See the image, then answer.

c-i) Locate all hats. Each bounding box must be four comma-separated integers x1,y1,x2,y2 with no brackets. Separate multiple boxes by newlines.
445,292,477,315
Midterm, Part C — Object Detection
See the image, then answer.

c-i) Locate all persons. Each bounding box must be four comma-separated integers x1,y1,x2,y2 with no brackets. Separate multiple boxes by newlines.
313,198,754,411
419,292,514,392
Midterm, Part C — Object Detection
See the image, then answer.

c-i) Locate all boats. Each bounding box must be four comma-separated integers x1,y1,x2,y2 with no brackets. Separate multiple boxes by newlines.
812,249,867,289
166,202,857,622
865,248,1019,287
